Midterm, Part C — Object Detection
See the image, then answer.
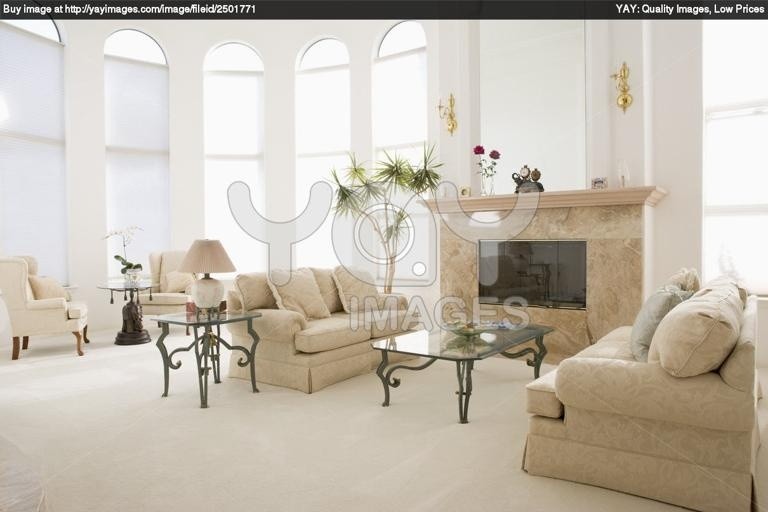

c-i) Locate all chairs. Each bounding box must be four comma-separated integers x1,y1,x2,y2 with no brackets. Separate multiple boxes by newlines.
0,252,90,360
134,248,195,316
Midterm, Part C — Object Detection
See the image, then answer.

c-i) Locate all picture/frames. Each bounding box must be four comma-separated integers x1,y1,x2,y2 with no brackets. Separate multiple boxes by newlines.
591,176,608,188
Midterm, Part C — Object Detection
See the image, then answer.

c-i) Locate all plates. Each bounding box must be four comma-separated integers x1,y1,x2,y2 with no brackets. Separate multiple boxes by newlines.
442,321,493,336
442,336,496,354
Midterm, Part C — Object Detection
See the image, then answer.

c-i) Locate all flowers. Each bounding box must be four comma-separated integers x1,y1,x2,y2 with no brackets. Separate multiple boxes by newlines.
473,144,487,175
101,223,144,274
486,150,501,177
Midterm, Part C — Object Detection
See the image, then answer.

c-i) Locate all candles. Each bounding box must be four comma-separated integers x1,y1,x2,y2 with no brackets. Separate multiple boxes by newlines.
438,98,441,104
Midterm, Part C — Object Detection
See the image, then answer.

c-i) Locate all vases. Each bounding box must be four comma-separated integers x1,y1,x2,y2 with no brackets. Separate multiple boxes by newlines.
126,267,140,281
489,176,495,196
480,173,488,197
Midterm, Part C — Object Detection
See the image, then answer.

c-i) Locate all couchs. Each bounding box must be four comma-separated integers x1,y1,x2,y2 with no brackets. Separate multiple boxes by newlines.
521,292,762,511
226,290,419,394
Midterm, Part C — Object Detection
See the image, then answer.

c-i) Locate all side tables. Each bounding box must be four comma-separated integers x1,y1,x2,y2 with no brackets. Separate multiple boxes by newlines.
151,313,262,407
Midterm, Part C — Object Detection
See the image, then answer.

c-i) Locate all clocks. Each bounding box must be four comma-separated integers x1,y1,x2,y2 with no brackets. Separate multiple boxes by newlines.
513,166,544,193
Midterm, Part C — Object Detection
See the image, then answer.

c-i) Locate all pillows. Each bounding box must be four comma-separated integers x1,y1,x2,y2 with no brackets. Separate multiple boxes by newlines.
647,281,745,379
159,250,205,293
329,265,384,313
165,271,198,293
703,239,747,310
233,272,279,316
310,267,344,313
629,269,700,363
267,267,331,321
28,273,71,302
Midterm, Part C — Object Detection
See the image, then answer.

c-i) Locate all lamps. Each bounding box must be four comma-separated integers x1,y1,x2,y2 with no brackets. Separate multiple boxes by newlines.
178,238,237,314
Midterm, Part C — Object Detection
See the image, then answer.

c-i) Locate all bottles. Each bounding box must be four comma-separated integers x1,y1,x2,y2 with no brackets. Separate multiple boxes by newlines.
618,158,630,189
